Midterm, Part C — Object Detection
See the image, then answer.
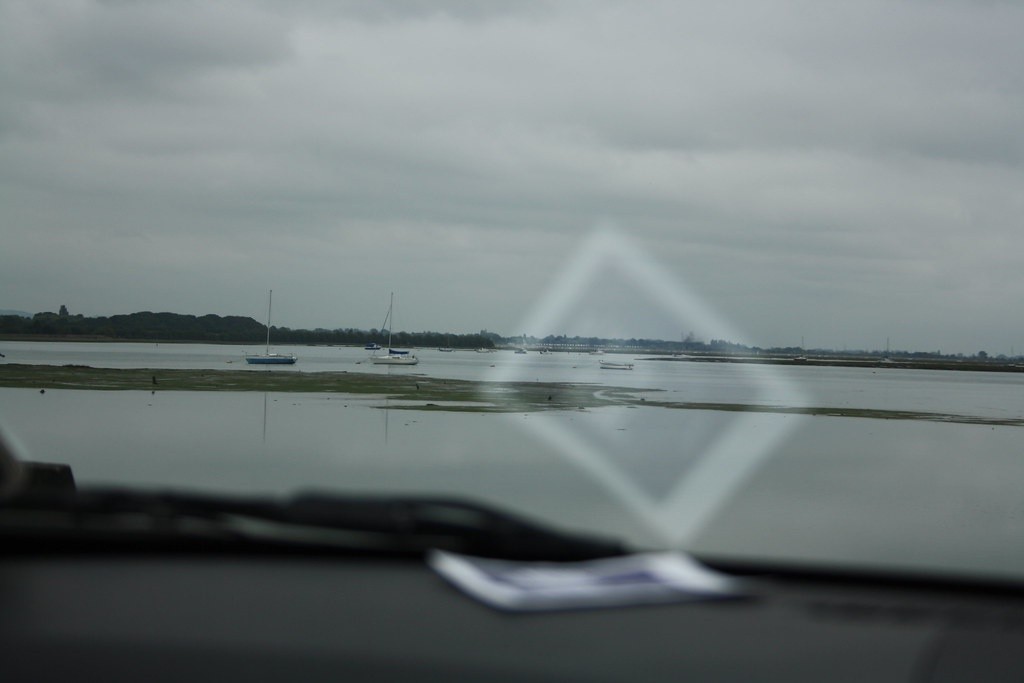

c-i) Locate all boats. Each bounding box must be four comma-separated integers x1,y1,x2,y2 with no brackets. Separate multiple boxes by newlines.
364,343,381,350
599,360,634,370
439,348,453,352
476,347,490,353
540,350,552,355
514,348,528,354
590,350,603,355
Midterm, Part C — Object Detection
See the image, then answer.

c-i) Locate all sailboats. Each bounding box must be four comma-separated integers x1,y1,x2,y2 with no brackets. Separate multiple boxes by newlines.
371,292,420,365
242,290,298,364
